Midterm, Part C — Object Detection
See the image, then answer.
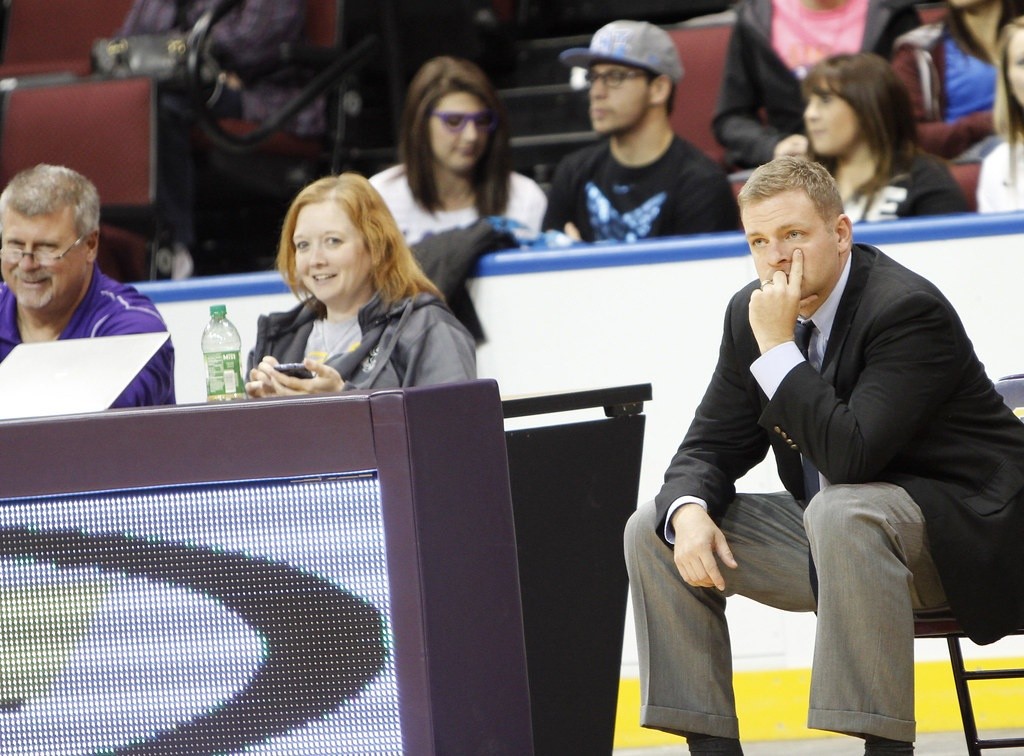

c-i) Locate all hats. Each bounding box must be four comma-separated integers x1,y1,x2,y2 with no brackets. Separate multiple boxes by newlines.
560,20,685,83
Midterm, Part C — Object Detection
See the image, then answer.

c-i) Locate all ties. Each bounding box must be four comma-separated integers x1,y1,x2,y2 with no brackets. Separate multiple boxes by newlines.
793,320,818,610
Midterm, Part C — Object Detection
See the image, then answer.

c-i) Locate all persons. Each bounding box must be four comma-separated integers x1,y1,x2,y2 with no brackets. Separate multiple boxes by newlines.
0,164,176,410
540,20,742,245
368,56,548,248
242,173,477,399
113,0,325,237
623,156,1024,756
710,0,1024,225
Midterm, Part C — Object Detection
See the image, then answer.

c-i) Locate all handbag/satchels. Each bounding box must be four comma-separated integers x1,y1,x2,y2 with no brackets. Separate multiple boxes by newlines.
93,4,230,108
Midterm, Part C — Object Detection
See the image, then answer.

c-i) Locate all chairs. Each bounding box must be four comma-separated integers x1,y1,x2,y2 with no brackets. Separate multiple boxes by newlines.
945,373,1024,756
0,72,164,284
662,21,751,169
0,0,144,75
200,0,349,259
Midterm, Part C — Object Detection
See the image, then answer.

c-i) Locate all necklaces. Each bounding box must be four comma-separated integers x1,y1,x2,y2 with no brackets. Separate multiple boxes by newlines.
321,319,357,361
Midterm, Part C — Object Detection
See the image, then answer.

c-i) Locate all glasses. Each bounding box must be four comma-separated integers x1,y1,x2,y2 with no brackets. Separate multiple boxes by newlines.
0,234,84,265
585,70,650,87
429,111,499,133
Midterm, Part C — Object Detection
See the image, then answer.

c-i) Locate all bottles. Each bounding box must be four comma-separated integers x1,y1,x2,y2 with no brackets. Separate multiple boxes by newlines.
201,303,247,404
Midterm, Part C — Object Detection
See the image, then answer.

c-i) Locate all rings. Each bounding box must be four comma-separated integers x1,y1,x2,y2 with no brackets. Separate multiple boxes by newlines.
761,281,773,290
